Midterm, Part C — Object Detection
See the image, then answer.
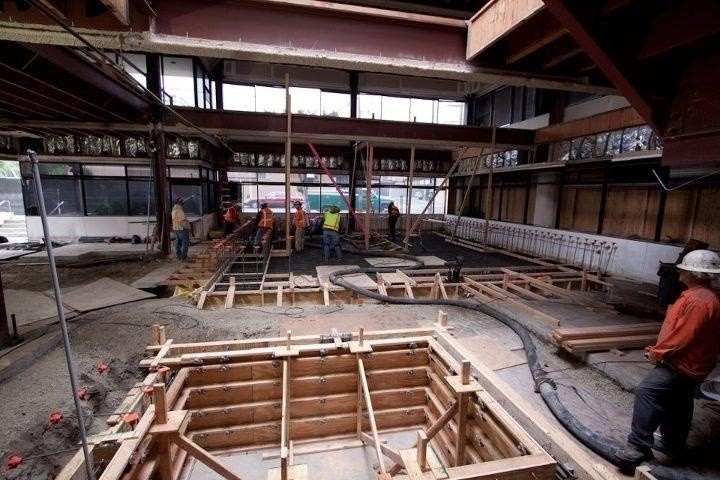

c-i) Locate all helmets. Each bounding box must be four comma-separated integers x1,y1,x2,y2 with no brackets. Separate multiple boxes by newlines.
293,201,302,205
676,249,720,274
330,206,340,214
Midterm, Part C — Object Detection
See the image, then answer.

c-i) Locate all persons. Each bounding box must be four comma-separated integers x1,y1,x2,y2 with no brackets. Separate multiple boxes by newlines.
322,206,344,260
224,202,242,238
171,197,190,261
388,203,401,241
614,249,720,463
293,201,306,252
254,203,273,246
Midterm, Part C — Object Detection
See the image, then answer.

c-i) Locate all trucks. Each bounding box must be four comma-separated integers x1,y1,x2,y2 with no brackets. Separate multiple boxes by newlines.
307,188,394,211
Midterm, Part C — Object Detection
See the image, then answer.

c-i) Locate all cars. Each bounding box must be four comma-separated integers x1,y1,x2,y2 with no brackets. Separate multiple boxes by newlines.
244,191,306,208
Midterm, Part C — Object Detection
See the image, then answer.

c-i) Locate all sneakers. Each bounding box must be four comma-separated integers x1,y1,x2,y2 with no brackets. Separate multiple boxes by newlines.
615,444,656,462
653,432,671,457
